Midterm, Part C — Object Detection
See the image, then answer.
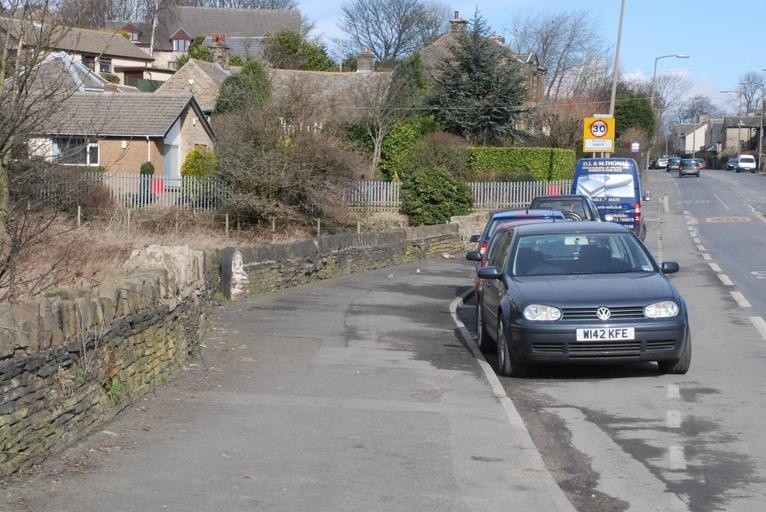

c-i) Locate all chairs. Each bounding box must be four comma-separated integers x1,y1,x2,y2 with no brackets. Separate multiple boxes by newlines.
517,249,546,276
580,246,613,271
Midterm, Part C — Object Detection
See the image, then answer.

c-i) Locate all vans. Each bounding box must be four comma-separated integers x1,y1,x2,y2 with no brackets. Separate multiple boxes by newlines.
574,156,652,242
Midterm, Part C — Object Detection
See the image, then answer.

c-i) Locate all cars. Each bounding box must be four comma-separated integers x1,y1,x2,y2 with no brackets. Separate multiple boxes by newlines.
679,159,700,177
667,157,681,173
726,157,738,171
695,159,705,169
736,154,757,174
472,221,693,376
471,206,567,306
532,194,602,227
655,157,667,169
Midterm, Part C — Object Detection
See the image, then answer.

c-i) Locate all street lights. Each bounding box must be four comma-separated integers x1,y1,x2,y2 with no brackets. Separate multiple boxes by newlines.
739,81,765,170
646,53,690,169
722,89,742,162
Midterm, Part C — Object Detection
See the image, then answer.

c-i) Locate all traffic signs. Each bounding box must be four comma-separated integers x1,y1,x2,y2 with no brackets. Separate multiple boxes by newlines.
584,118,614,152
